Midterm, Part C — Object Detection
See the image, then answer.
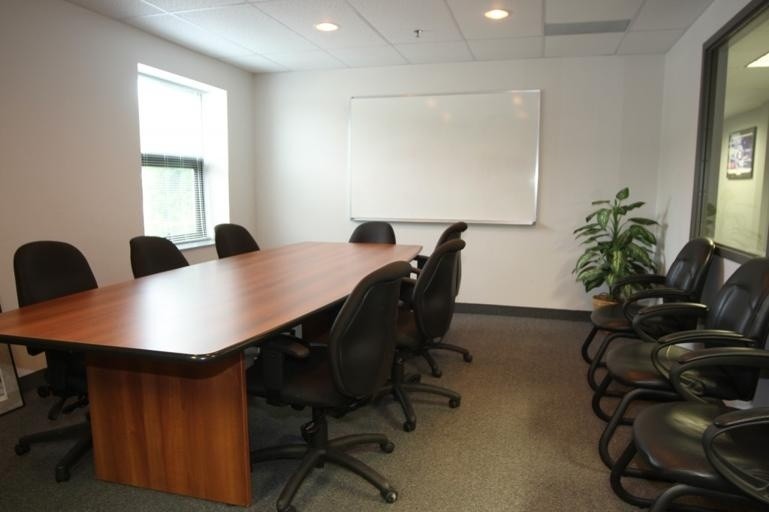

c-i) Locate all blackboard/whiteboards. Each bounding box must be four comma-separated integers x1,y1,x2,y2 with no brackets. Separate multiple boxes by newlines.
348,89,542,225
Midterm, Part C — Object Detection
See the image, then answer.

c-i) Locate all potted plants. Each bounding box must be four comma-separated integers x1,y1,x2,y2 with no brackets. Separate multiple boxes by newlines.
571,187,659,310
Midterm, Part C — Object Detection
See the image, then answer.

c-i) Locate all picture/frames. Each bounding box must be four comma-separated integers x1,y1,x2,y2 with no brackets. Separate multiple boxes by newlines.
726,126,756,180
